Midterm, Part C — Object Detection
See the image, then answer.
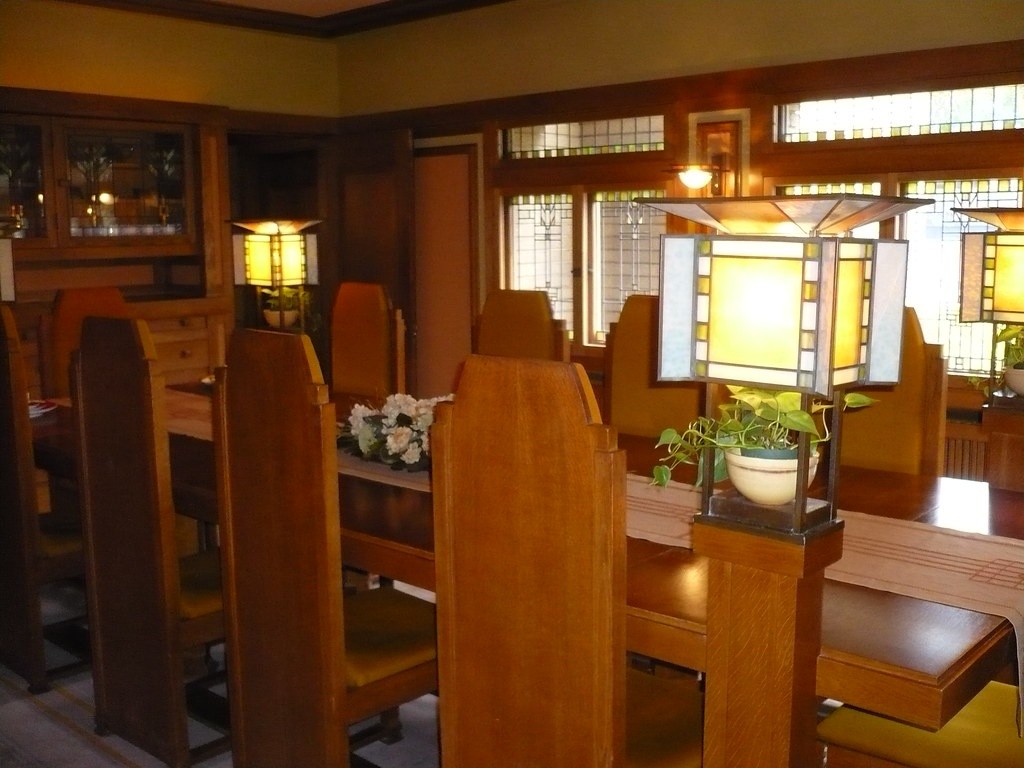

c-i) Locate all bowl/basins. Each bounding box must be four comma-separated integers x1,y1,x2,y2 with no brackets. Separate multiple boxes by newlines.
28,400,57,418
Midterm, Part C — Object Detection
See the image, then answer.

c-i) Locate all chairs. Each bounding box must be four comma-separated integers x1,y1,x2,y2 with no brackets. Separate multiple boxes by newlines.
74,313,230,767
471,291,571,363
817,679,1024,767
199,328,440,768
330,280,406,399
0,305,197,695
601,294,707,485
431,355,704,767
39,286,141,394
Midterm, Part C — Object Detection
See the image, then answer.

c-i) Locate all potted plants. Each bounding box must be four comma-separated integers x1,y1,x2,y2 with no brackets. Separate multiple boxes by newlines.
652,387,884,508
261,286,308,328
996,325,1024,398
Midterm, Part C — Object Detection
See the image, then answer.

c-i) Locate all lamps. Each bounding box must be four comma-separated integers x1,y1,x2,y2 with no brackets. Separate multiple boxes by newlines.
629,194,936,533
678,164,713,189
950,206,1024,324
223,215,323,286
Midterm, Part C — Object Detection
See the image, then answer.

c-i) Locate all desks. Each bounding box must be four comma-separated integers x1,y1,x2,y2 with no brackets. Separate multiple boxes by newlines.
34,381,1024,735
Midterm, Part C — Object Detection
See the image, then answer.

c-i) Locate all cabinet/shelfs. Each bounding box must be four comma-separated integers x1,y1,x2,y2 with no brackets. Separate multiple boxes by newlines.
0,112,203,260
